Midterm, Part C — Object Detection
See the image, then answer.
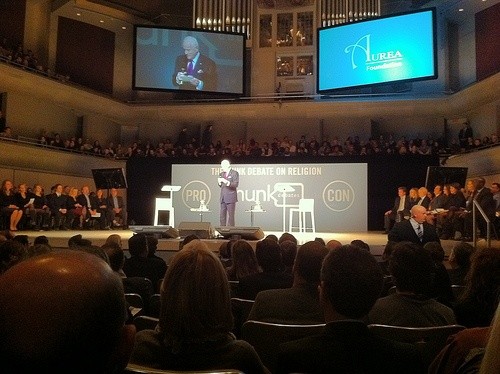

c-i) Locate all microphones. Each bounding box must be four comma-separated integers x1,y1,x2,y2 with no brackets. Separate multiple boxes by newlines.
219,173,223,183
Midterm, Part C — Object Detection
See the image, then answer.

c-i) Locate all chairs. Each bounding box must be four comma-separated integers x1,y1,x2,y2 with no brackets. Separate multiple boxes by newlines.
120,277,469,374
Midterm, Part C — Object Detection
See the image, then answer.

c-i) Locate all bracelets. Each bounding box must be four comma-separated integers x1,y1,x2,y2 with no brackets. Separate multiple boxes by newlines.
193,79,199,87
226,180,228,186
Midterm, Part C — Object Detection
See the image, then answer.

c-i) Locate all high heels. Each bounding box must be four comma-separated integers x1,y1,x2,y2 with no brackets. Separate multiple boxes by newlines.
9,227,18,232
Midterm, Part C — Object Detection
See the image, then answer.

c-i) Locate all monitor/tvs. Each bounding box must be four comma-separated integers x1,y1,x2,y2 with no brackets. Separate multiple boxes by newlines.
134,24,247,96
315,6,435,96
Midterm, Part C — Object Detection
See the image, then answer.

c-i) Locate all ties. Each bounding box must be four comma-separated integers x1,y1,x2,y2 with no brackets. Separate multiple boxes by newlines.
417,223,423,242
223,172,226,178
187,60,193,75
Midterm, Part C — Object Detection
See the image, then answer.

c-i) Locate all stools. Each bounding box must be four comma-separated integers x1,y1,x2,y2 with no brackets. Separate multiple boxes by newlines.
289,198,315,233
154,198,174,226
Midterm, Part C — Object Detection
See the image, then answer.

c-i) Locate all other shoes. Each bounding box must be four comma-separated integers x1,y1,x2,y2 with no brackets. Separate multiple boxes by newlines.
440,233,451,240
382,230,389,234
30,222,121,232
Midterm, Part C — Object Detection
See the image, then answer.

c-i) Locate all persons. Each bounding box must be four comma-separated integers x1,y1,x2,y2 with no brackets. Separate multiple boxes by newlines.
0,179,127,232
0,36,71,83
382,177,500,241
37,124,500,160
0,233,500,374
277,82,283,103
172,35,217,91
387,205,441,247
0,110,14,142
218,159,239,227
